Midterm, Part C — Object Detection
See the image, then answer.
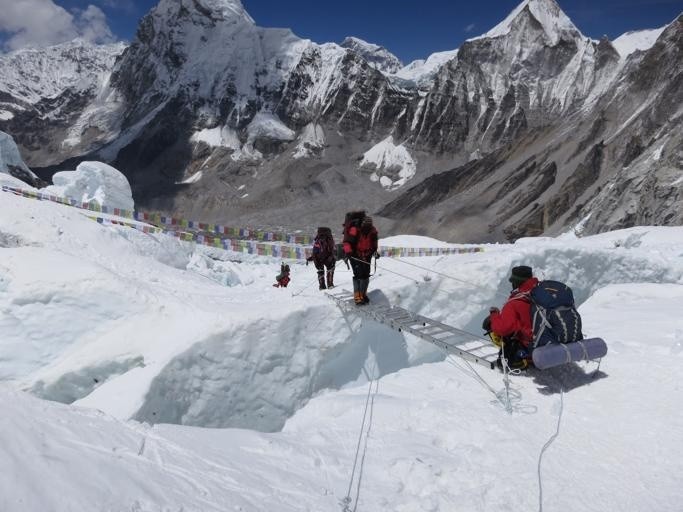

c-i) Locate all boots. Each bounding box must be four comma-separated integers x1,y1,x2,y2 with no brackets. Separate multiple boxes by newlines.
317,270,328,291
361,278,371,303
350,276,364,306
325,270,336,289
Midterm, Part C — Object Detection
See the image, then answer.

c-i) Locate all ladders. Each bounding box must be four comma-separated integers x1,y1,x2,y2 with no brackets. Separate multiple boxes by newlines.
324,288,502,370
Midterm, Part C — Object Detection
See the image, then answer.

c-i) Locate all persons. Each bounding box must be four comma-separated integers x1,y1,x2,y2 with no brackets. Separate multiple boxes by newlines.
313,234,335,290
343,217,380,305
277,260,290,287
490,266,540,373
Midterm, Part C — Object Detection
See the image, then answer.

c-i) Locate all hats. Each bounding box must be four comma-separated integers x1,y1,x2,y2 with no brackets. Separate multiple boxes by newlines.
509,265,534,284
362,216,374,224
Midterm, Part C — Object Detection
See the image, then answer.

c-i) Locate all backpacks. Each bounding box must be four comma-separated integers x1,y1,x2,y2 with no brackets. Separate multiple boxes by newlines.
529,279,583,351
342,210,366,236
